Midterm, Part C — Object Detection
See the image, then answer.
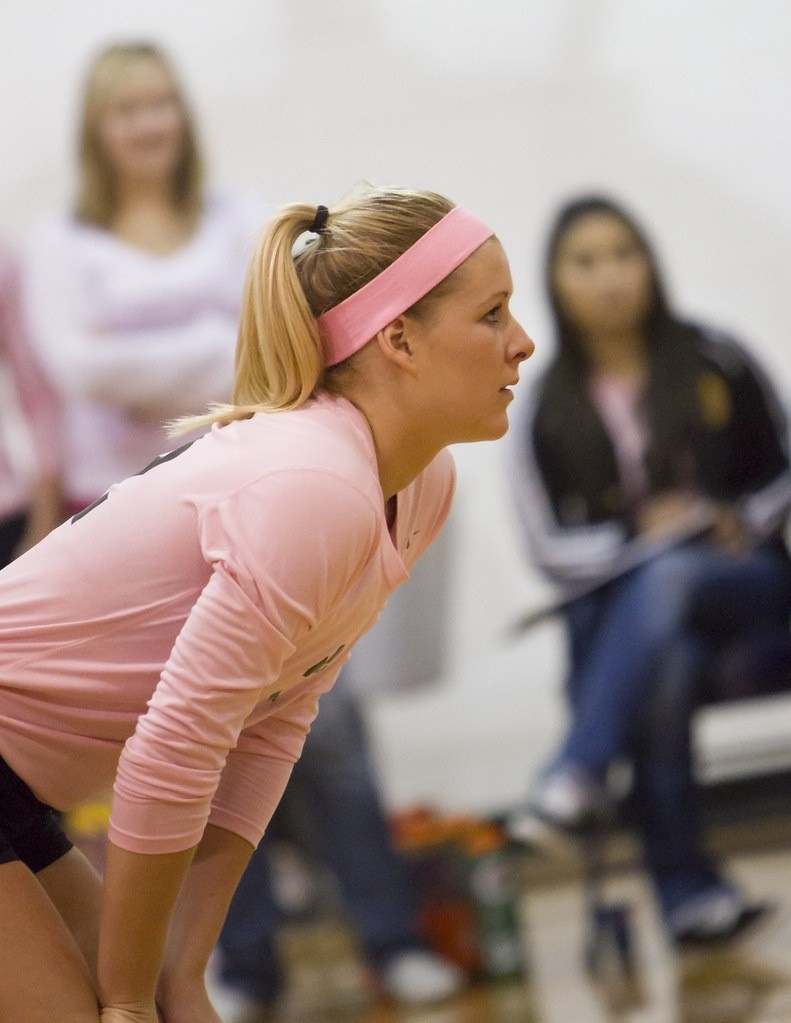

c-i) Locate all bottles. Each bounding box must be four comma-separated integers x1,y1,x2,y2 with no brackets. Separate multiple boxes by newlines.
462,839,527,990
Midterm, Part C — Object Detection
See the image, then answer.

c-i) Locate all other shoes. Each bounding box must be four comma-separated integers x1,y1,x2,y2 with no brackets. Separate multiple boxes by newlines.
508,772,603,850
205,946,279,1021
379,952,461,1006
661,890,750,946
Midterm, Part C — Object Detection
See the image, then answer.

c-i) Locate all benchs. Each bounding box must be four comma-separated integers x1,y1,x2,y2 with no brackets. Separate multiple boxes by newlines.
389,697,791,984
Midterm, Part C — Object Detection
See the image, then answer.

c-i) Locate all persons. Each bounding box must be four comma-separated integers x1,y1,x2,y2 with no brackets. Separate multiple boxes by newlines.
0,186,539,1023
0,38,458,1023
504,195,791,970
